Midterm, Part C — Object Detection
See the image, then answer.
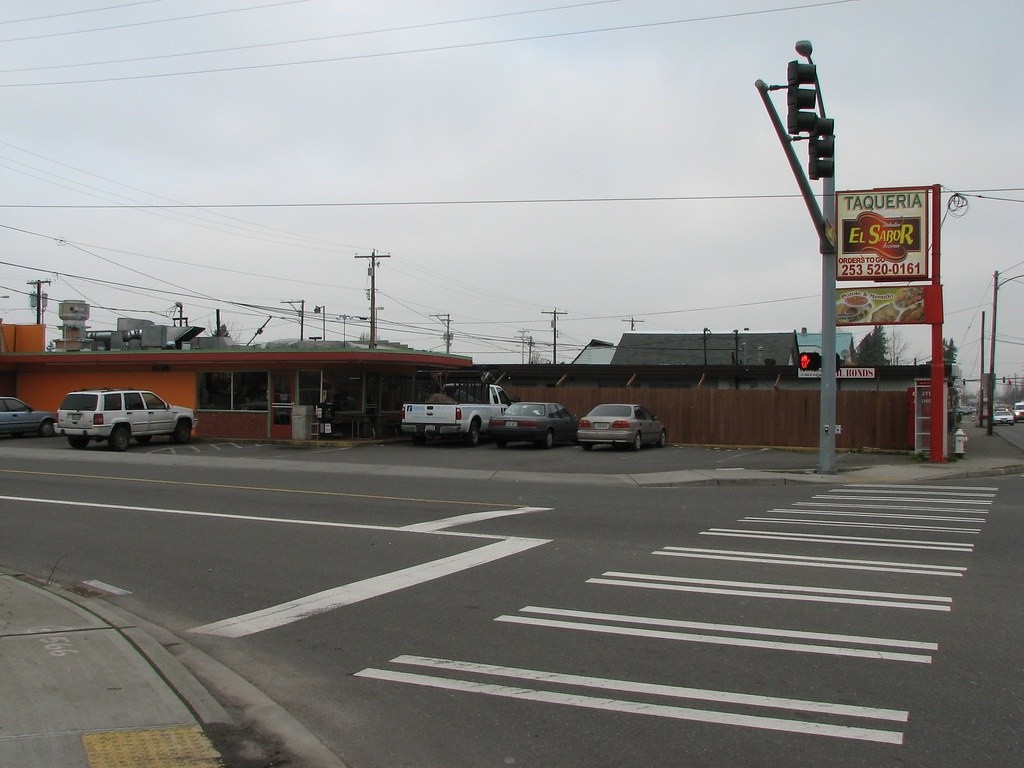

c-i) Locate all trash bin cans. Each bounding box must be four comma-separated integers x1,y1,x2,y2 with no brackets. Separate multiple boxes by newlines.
291,404,314,440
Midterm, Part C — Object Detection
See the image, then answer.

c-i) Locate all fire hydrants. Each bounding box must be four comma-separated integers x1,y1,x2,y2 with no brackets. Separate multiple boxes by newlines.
953,429,969,455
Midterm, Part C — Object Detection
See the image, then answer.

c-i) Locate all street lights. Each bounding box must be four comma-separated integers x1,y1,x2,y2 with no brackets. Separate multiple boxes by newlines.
369,306,383,344
795,40,840,471
314,305,325,342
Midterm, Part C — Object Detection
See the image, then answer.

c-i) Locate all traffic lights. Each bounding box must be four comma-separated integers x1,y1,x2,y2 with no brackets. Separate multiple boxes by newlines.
787,61,820,133
1009,380,1012,385
799,352,822,371
809,118,834,180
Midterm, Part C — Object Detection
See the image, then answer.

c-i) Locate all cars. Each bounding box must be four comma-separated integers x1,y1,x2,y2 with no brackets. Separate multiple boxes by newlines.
956,405,977,415
488,401,577,449
577,403,666,451
992,401,1024,426
0,397,58,437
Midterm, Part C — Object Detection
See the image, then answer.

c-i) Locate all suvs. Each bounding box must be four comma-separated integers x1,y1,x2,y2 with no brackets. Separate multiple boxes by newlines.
52,387,198,452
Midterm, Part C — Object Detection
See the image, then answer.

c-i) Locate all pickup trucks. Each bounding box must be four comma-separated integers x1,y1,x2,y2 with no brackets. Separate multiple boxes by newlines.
400,382,513,447
